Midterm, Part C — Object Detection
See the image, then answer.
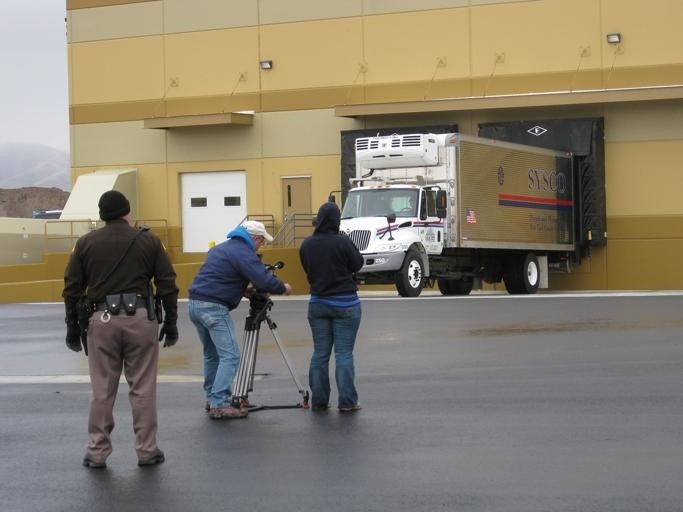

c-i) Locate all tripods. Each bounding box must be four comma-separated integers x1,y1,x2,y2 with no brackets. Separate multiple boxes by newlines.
231,316,310,409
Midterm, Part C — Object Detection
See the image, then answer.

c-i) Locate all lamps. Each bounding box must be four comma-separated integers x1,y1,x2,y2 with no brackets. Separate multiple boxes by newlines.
260,60,272,69
607,33,620,43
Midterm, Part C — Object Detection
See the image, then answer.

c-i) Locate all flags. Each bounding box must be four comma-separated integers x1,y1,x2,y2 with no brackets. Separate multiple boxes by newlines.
465,209,478,224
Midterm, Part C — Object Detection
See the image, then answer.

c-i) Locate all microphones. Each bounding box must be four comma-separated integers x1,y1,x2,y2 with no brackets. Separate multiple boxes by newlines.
276,261,284,269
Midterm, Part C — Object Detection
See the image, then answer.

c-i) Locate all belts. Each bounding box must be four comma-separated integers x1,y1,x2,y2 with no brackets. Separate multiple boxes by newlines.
92,298,157,312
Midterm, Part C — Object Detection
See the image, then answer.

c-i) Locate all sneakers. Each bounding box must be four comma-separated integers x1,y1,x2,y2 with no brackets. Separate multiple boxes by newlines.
340,402,360,411
83,459,106,468
138,451,164,465
205,398,248,418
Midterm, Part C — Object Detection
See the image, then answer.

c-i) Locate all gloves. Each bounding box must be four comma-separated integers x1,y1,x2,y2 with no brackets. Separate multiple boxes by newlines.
159,320,178,348
66,324,82,352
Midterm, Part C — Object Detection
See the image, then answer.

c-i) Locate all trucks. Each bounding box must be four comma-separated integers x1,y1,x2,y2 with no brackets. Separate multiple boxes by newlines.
337,133,576,297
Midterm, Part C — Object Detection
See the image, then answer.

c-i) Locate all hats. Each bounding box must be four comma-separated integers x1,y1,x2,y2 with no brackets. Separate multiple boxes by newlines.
242,221,274,242
98,191,130,220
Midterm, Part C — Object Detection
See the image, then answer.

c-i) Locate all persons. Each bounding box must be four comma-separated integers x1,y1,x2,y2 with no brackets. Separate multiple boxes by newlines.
188,220,292,421
61,189,180,469
297,201,365,414
